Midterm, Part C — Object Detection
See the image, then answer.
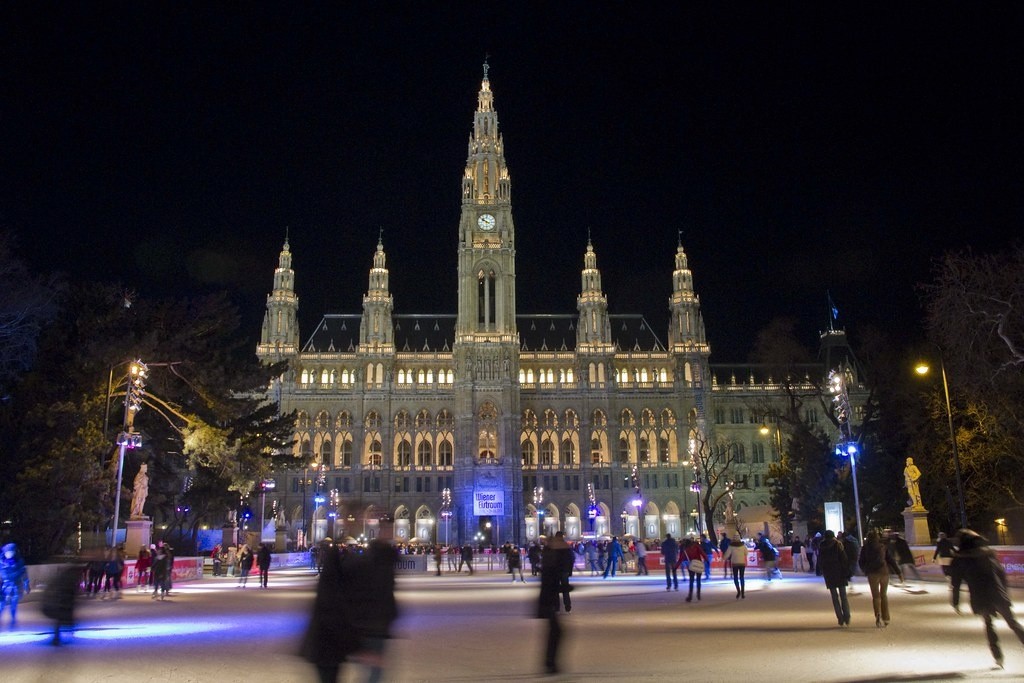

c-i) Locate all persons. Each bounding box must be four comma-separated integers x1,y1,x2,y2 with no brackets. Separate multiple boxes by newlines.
815,530,853,628
86,547,125,599
571,535,661,579
904,457,922,508
673,538,709,602
858,532,904,627
533,531,575,675
299,536,402,683
933,532,960,588
0,543,31,630
837,531,920,582
131,463,149,514
720,531,748,599
504,541,541,583
755,532,782,581
661,532,719,591
209,542,271,590
397,540,475,576
136,541,175,600
37,563,85,647
944,528,1024,671
792,532,825,573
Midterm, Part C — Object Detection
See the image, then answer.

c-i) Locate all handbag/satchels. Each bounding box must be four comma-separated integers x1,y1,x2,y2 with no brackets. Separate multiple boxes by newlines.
689,560,704,573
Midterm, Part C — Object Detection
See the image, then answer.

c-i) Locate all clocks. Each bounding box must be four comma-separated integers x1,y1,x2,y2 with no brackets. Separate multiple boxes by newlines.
478,213,497,232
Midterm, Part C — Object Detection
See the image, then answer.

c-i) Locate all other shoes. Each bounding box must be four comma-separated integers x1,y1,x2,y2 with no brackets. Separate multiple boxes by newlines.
884,618,890,626
686,597,692,602
674,587,678,589
875,613,882,627
996,656,1004,669
736,592,741,599
666,586,671,589
742,595,745,599
697,594,701,600
837,617,844,625
845,616,850,626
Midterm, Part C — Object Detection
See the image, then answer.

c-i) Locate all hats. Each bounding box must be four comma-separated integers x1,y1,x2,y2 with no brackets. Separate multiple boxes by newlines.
733,531,741,541
